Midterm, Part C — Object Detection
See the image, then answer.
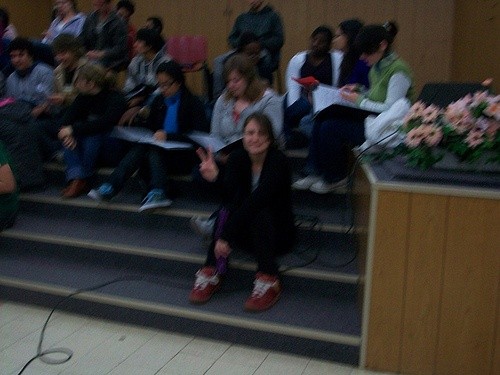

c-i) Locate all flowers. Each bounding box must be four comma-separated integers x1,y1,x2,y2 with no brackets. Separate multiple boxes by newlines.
397,89,500,172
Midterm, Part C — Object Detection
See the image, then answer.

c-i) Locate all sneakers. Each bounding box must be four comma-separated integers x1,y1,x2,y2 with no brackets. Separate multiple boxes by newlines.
309,177,349,194
245,271,281,311
188,268,222,303
293,175,321,190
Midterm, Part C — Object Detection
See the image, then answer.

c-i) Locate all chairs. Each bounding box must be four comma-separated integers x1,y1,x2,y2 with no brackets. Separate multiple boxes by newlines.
412,82,491,112
166,35,208,65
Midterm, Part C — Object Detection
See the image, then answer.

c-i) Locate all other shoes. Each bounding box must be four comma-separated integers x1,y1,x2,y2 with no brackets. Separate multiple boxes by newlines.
138,188,172,215
89,182,115,202
193,217,215,240
64,179,84,198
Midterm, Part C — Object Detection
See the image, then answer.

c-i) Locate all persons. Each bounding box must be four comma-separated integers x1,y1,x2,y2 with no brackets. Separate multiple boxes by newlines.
285,19,416,191
0,0,203,212
211,0,285,100
202,54,284,230
190,111,292,311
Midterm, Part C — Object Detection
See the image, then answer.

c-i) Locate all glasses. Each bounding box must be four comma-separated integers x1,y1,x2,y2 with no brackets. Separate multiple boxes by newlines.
158,78,177,90
